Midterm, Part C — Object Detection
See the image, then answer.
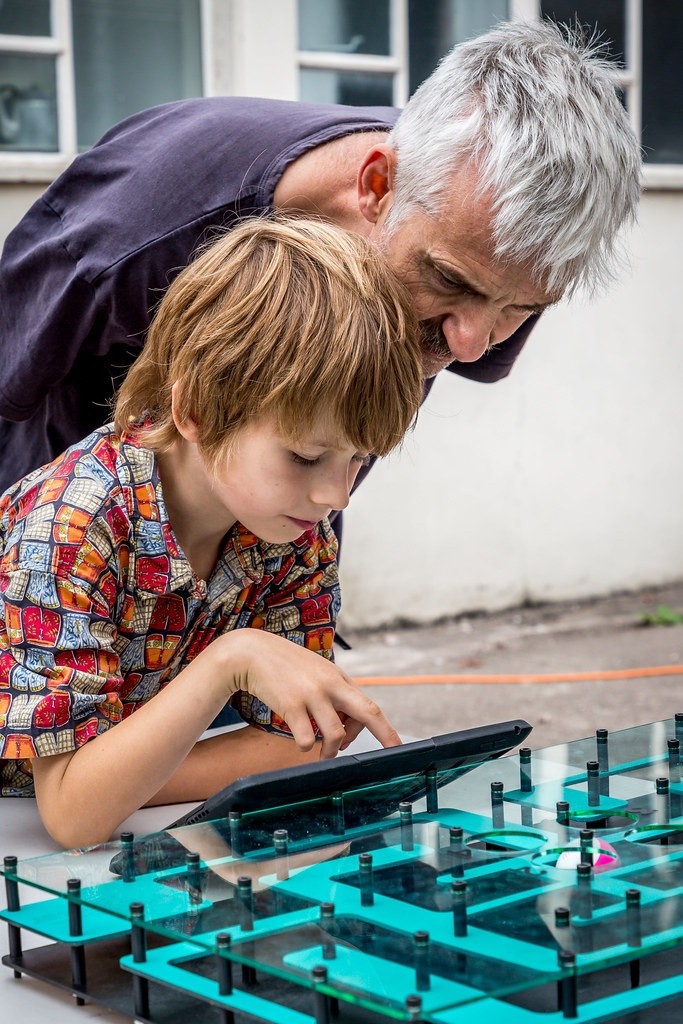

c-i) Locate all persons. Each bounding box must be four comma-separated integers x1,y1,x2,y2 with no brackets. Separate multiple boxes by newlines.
2,25,640,514
2,216,416,854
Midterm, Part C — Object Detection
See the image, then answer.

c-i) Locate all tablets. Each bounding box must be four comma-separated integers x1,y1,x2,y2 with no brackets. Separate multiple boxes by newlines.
109,720,533,877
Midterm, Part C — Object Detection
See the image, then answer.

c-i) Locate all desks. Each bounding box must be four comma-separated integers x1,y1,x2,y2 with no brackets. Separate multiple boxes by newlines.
0,715,661,1024
